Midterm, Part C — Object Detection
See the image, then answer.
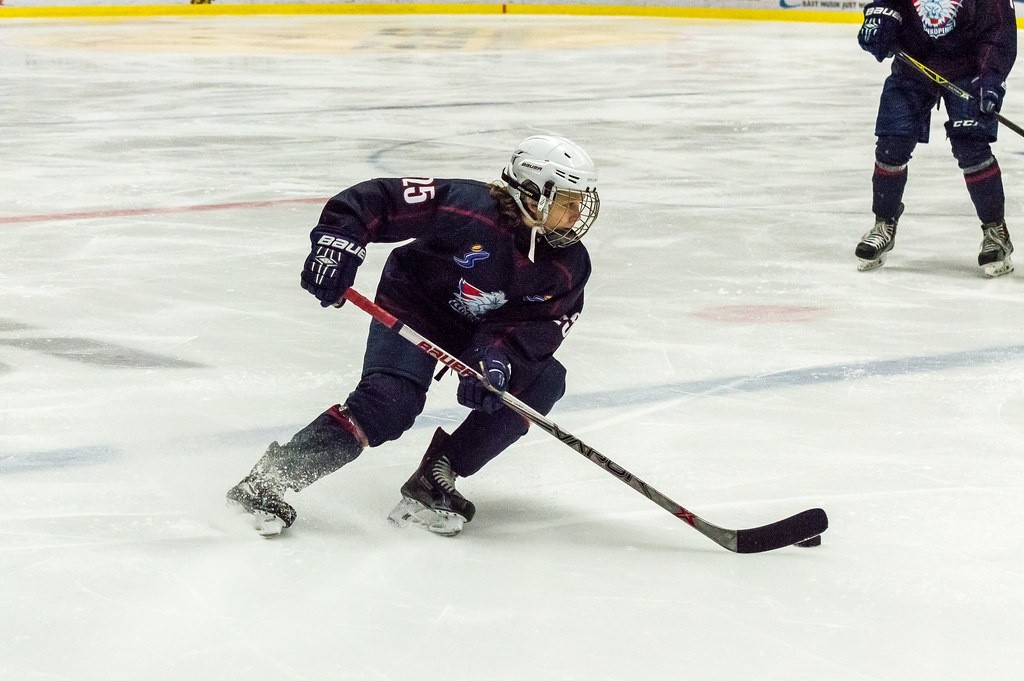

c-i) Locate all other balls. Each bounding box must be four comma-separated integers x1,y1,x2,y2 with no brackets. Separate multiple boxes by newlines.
793,535,822,548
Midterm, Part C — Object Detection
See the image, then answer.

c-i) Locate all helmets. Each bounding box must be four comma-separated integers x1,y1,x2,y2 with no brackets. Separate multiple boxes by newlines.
506,136,597,228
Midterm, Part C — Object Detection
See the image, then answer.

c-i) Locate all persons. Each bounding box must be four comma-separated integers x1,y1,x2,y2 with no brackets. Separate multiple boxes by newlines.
854,0,1017,281
219,135,599,538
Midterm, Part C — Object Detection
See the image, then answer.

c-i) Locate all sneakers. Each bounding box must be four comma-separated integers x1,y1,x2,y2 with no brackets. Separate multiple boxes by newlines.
225,440,297,537
854,202,904,271
977,218,1014,277
387,427,475,536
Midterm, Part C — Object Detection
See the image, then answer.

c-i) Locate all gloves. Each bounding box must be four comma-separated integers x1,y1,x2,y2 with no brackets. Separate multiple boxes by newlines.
300,226,367,310
950,80,1006,137
457,346,511,415
857,2,903,63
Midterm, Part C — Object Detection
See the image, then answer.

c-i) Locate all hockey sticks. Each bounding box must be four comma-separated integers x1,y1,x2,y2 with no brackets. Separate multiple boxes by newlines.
342,284,829,554
889,45,1023,137
